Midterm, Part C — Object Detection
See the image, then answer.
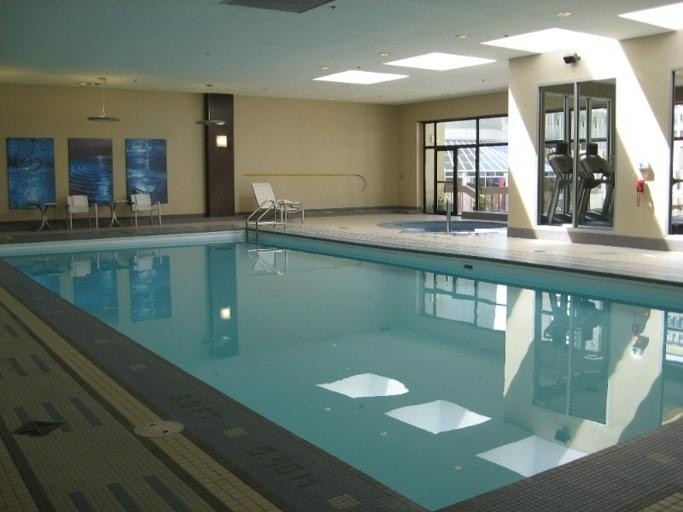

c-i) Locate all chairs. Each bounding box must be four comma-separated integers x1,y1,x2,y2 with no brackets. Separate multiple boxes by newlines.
251,182,305,229
63,192,162,229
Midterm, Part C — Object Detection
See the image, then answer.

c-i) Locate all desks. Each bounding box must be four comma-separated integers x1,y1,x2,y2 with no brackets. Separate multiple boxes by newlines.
26,201,61,232
